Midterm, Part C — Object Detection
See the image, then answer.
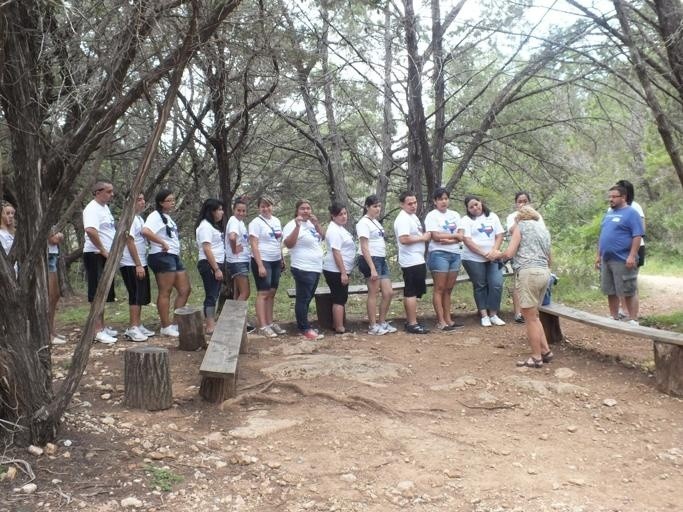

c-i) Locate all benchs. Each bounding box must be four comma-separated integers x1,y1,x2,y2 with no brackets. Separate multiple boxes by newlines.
286,266,514,330
198,299,250,404
537,302,683,401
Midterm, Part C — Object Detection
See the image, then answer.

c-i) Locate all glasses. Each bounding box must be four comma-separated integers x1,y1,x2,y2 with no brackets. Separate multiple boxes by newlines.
607,195,621,198
163,199,175,202
92,188,105,196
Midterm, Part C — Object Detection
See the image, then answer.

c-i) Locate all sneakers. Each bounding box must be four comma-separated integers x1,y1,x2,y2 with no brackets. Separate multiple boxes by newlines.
490,315,505,326
380,321,397,333
367,324,389,336
52,337,67,344
449,321,464,328
404,321,429,332
95,324,180,343
481,316,491,326
514,313,522,321
246,322,324,340
617,312,627,319
406,324,427,334
435,324,455,332
627,319,639,326
57,334,66,339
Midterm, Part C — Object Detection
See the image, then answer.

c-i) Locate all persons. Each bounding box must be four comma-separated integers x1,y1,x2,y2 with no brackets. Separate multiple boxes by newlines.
355,195,398,336
594,180,646,326
196,193,356,338
394,191,504,334
1,200,65,344
82,181,191,344
497,192,554,367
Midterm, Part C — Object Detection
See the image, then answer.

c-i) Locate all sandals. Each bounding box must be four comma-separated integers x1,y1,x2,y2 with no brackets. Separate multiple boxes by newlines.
516,355,542,367
541,351,552,362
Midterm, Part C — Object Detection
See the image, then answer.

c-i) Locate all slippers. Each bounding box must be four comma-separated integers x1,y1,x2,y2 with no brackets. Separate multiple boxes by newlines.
336,327,354,334
